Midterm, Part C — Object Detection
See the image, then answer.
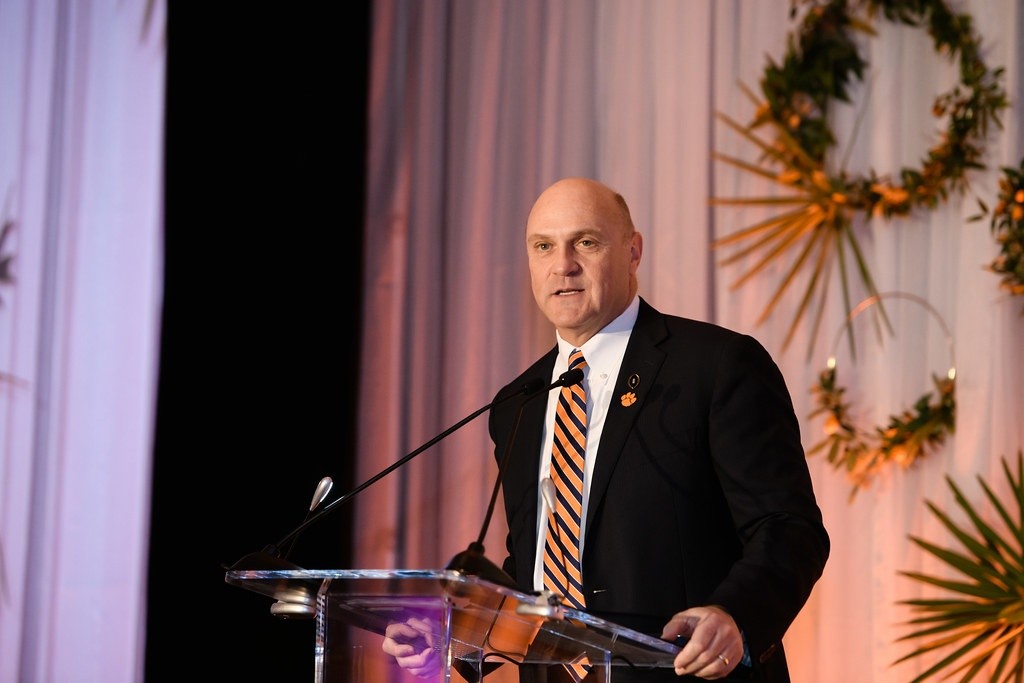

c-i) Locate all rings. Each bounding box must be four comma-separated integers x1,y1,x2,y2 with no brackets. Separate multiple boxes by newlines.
717,654,732,666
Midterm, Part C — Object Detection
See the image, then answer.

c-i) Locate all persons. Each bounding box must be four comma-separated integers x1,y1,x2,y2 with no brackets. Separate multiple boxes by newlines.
382,179,832,683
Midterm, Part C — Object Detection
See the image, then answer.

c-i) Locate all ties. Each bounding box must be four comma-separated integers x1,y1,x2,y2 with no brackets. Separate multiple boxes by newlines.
543,348,594,683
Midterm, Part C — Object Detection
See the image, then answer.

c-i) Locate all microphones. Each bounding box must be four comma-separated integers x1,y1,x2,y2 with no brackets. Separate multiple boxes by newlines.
228,377,545,573
439,369,584,597
